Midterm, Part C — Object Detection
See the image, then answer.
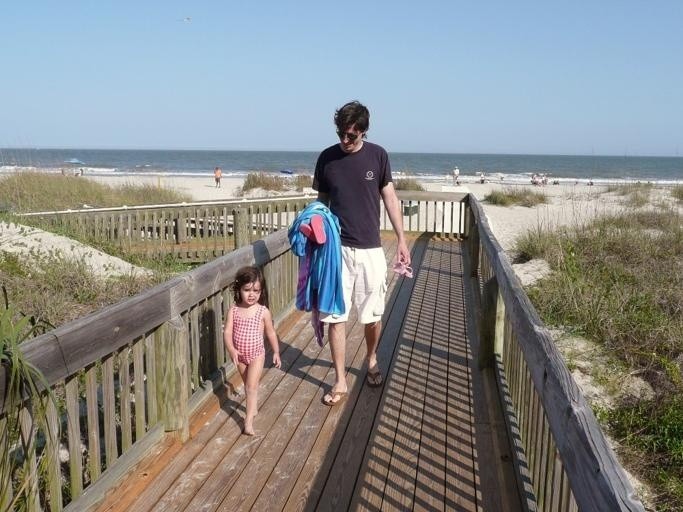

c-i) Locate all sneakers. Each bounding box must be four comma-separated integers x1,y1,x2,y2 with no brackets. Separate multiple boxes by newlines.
300,224,316,242
311,215,327,244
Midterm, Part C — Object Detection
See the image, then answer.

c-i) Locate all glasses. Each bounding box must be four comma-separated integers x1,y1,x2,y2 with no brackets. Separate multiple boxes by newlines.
336,131,361,139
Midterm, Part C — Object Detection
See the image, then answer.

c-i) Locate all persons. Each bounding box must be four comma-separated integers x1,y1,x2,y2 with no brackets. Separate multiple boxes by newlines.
451,166,459,186
311,100,414,407
214,167,222,188
224,266,282,437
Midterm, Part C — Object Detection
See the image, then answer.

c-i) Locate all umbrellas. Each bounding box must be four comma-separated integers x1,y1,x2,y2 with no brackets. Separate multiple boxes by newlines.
63,158,84,177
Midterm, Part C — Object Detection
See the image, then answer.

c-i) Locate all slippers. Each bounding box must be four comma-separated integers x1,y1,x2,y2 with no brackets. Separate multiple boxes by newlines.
393,263,404,276
367,372,383,388
403,267,413,279
323,390,349,405
